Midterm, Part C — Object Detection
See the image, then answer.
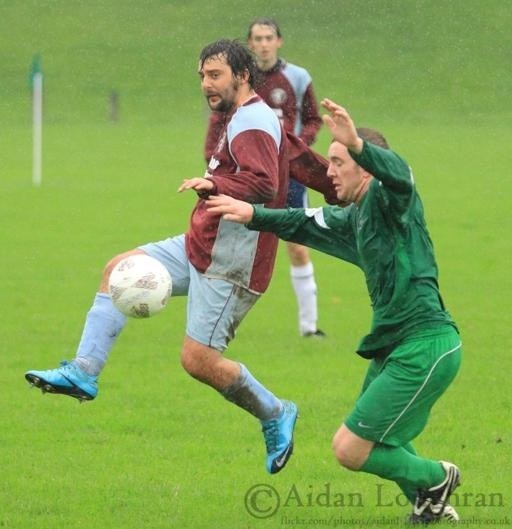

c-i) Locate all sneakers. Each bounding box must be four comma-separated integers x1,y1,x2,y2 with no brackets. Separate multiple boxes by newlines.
261,399,298,473
408,460,462,525
25,358,98,401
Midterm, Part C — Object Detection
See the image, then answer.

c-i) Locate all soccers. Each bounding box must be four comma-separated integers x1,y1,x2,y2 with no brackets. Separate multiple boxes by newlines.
109,254,172,318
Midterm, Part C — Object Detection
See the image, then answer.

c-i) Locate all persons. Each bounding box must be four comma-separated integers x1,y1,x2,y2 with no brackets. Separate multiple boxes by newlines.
24,37,299,474
201,15,327,336
205,97,466,526
